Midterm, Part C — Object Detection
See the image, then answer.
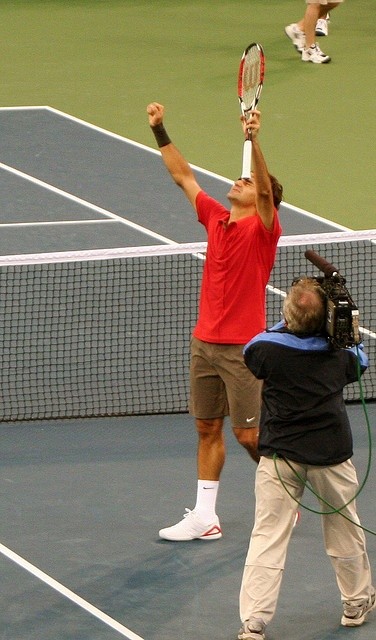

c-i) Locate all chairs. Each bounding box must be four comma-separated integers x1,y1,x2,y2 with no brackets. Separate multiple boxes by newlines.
158,507,223,541
315,19,328,36
238,616,267,640
284,23,305,54
301,43,331,64
341,593,376,627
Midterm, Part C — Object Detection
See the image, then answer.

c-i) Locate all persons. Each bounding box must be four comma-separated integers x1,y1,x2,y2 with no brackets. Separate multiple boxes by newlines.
315,10,331,36
144,101,284,544
238,274,376,639
285,0,343,67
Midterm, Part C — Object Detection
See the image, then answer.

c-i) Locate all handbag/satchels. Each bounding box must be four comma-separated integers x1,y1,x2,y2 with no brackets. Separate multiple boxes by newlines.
149,122,172,149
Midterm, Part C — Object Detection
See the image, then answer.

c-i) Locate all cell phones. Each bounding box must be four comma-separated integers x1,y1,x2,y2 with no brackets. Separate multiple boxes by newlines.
292,251,362,347
